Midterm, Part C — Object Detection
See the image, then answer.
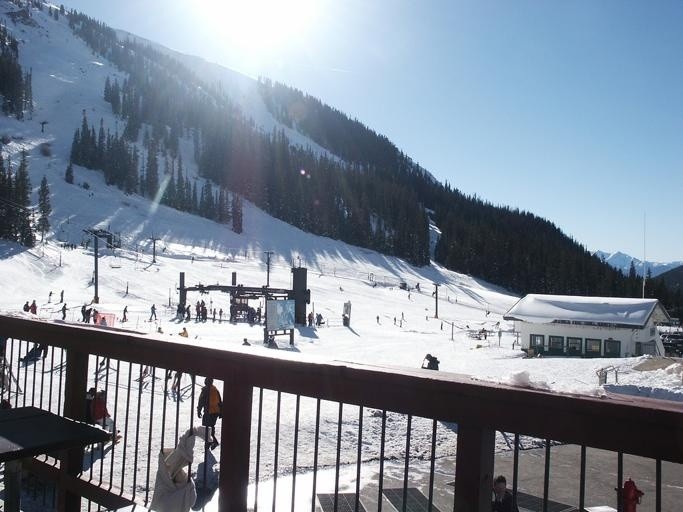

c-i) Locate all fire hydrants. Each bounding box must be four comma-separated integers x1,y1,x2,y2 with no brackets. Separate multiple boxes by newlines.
615,478,645,511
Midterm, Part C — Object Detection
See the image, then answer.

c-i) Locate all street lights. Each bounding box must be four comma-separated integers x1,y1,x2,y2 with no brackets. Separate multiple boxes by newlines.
149,238,161,263
432,283,441,318
264,251,274,287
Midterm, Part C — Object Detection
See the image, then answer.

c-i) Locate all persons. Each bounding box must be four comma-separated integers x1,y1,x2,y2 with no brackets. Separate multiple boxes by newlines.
211,306,261,322
268,335,276,346
24,288,108,325
490,475,519,512
308,313,322,326
89,388,122,445
421,353,439,370
35,344,48,358
195,377,222,449
85,387,98,425
242,338,250,346
149,300,206,338
122,306,128,322
